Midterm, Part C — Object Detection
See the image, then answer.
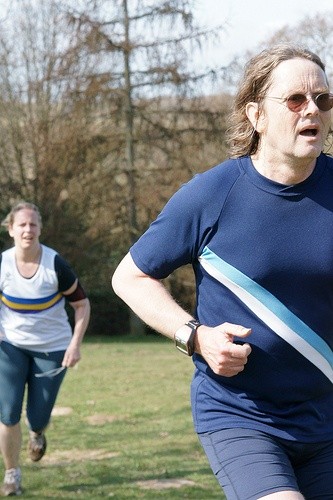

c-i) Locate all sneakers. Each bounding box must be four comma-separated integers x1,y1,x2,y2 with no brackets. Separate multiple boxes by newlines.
0,469,21,496
28,432,47,462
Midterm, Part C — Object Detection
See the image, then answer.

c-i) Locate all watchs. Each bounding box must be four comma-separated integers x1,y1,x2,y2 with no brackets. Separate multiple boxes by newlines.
174,319,202,356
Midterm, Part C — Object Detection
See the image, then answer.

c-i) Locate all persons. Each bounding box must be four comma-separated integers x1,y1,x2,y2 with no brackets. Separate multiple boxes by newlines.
0,201,89,497
112,48,333,500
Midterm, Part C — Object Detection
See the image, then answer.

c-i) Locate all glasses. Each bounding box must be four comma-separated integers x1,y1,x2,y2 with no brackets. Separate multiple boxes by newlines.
255,92,333,113
